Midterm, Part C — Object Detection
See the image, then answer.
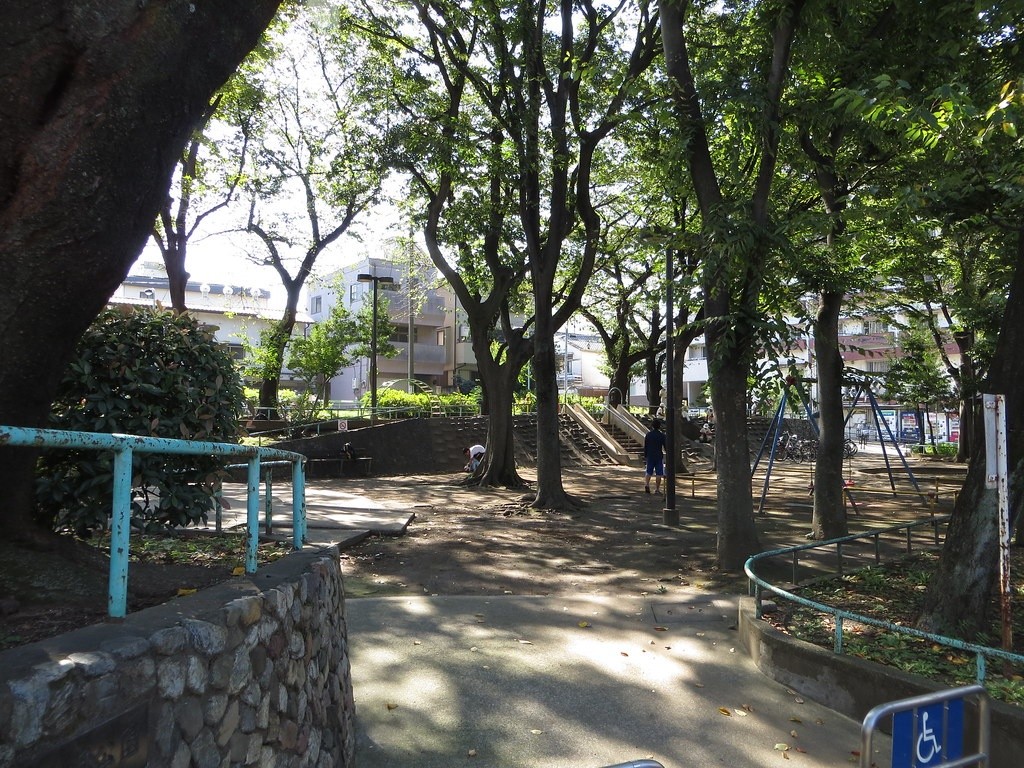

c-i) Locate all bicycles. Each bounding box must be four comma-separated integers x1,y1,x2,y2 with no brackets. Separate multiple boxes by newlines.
774,430,858,464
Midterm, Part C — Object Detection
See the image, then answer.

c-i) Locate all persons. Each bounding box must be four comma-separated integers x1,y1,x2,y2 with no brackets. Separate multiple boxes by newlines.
656,385,667,419
681,402,691,421
643,420,666,495
700,423,711,440
701,408,713,422
858,421,868,444
463,445,485,472
296,446,304,455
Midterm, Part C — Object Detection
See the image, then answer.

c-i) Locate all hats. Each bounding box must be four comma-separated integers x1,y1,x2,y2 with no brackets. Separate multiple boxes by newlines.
703,424,708,429
660,403,664,406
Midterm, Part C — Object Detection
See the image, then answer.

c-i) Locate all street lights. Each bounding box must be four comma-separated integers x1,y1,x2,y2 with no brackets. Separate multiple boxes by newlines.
357,274,394,419
558,329,576,391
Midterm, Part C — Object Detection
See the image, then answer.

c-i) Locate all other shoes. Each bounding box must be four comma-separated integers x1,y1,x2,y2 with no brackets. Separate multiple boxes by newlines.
645,485,650,493
654,490,663,496
